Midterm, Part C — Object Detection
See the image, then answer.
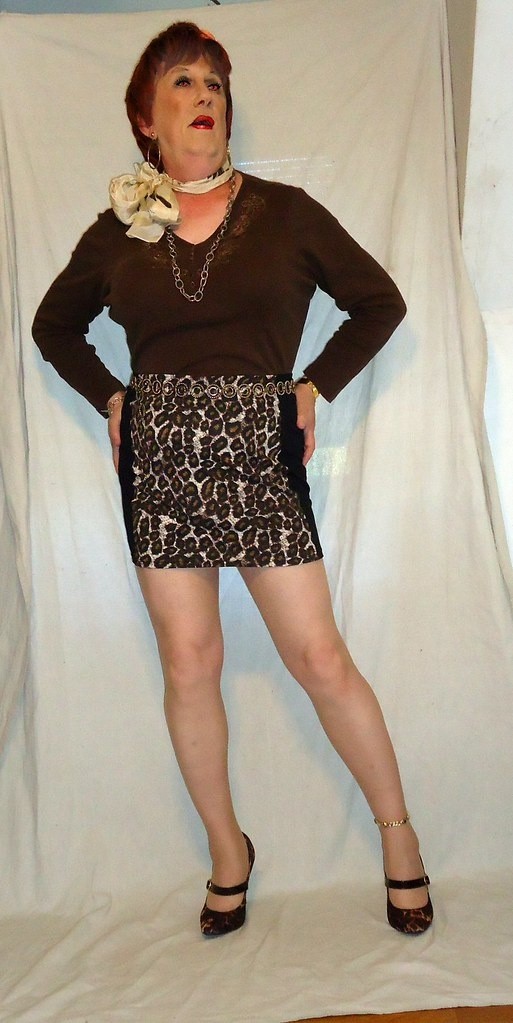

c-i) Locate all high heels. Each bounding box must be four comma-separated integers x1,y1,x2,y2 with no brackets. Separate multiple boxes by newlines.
382,848,434,935
200,831,255,936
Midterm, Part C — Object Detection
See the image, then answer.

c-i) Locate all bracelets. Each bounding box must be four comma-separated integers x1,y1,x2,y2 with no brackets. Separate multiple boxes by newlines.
107,396,124,415
298,378,319,398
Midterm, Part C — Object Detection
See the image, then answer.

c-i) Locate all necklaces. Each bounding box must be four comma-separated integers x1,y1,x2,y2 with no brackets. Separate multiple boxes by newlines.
165,175,236,302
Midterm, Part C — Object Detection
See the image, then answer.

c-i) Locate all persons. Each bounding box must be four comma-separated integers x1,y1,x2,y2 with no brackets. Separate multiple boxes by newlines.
28,20,432,936
